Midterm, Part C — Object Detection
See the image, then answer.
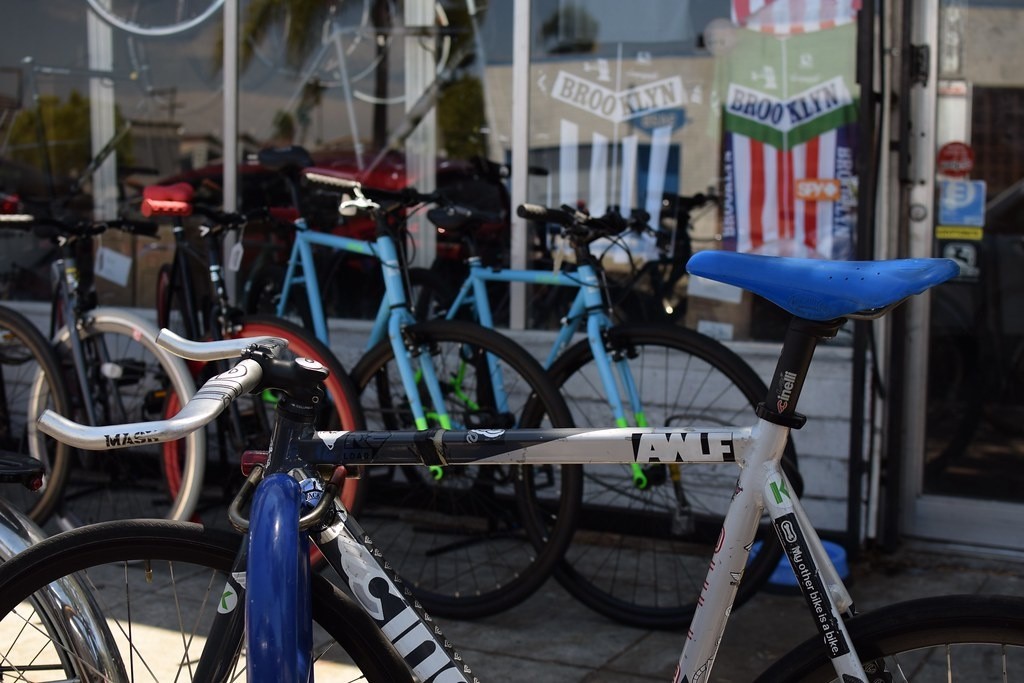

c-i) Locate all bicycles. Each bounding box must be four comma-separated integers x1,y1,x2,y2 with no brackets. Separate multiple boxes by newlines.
0,89,1024,683
